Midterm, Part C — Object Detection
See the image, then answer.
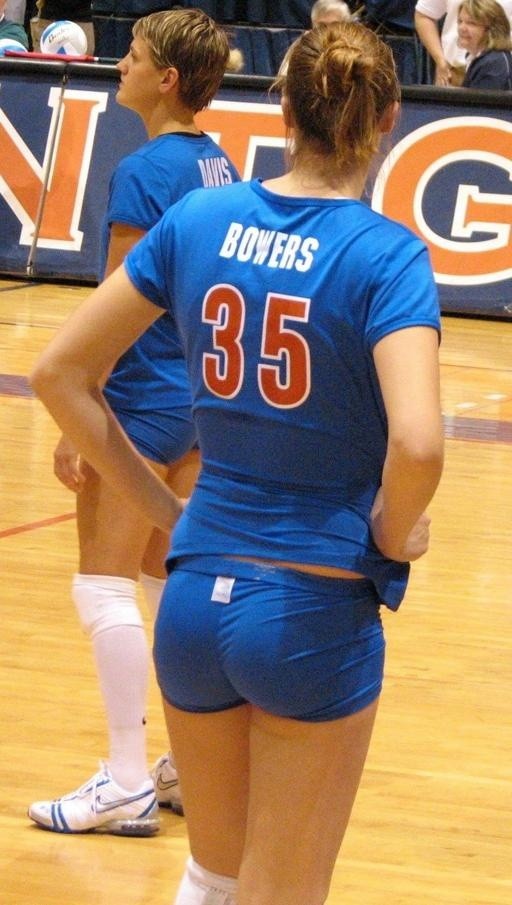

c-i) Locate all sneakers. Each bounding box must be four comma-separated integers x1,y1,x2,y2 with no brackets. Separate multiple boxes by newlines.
148,749,183,816
27,757,160,837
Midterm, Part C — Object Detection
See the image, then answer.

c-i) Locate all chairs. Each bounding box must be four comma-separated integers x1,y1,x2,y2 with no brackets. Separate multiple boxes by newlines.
22,0,448,96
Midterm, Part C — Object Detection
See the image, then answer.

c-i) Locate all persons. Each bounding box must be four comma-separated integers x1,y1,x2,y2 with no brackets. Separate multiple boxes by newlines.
0,0,29,55
311,0,352,29
30,18,449,905
414,0,512,88
456,0,512,90
27,9,240,838
